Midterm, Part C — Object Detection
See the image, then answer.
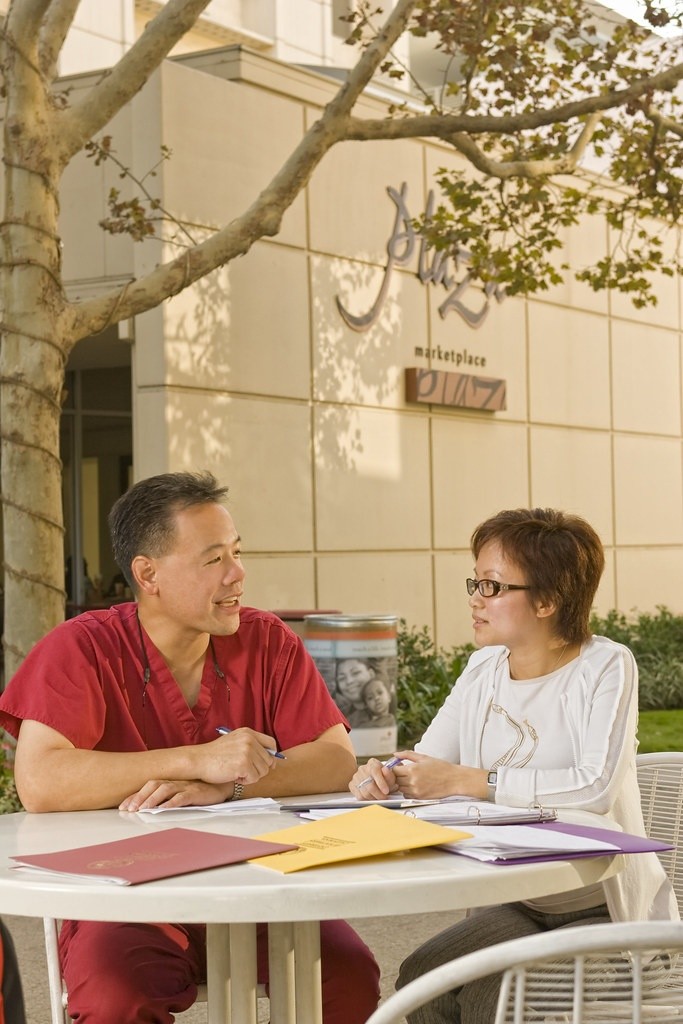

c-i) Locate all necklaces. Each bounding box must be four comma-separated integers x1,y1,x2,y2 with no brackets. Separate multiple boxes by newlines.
553,645,568,671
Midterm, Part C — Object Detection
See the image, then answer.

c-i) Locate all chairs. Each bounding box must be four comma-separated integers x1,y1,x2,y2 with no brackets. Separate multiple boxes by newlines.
44,919,267,1024
357,915,682,1024
635,750,682,923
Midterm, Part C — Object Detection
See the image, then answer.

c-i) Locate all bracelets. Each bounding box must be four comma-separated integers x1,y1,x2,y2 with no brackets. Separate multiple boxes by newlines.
232,782,245,801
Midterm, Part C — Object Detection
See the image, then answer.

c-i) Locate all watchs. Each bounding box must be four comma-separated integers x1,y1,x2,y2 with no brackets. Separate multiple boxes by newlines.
486,771,498,800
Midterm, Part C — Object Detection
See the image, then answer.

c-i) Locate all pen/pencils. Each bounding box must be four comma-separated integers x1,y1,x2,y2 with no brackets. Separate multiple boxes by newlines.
357,750,414,789
214,727,287,760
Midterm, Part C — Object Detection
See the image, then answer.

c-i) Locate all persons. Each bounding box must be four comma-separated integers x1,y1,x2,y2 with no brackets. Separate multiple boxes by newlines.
349,509,683,1024
333,658,396,729
0,469,359,1024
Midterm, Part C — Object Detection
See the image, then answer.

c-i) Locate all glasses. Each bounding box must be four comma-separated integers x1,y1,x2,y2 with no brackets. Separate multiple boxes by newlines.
466,578,530,598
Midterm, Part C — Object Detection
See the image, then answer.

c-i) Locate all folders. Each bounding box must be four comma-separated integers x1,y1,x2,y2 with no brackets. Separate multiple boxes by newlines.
291,793,676,867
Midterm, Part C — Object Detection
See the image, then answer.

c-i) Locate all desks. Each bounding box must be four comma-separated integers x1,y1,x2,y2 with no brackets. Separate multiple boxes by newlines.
0,793,625,1024
